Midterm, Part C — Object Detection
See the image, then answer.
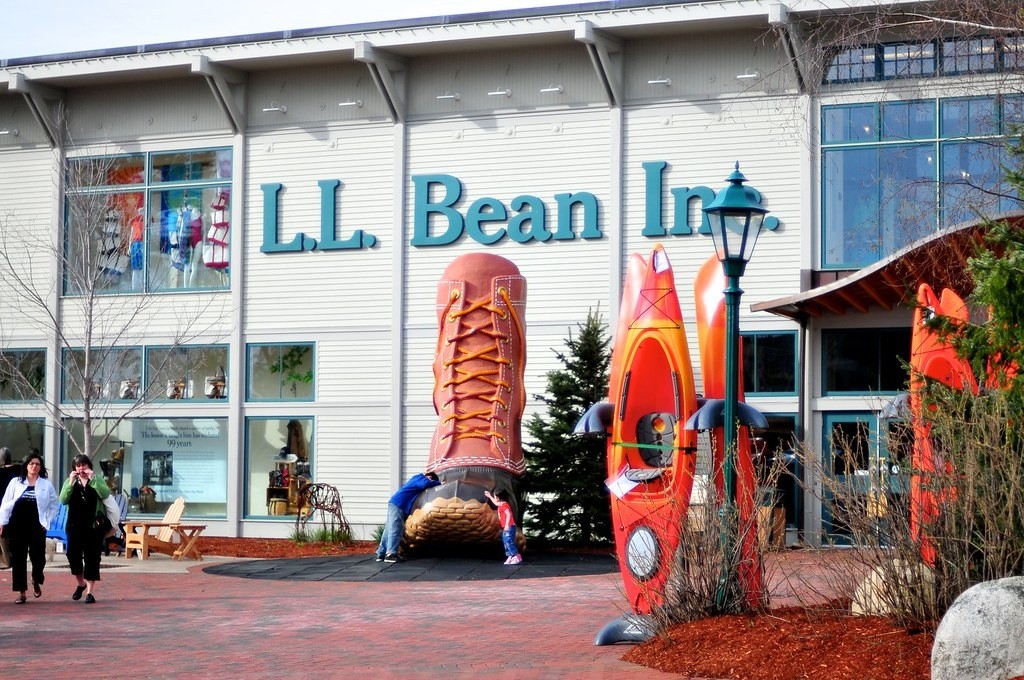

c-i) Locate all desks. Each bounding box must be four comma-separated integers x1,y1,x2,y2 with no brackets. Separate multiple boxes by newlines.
171,524,208,562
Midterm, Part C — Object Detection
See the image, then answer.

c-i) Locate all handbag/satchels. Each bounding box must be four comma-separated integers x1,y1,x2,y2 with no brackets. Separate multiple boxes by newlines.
0,535,11,569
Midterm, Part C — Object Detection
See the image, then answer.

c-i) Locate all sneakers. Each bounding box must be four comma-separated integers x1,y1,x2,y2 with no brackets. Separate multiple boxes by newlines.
504,556,512,566
376,555,383,561
510,554,522,565
384,554,398,562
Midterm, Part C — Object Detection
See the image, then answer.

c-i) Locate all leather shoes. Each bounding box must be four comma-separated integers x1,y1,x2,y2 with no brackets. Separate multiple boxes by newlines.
85,594,95,603
72,584,87,600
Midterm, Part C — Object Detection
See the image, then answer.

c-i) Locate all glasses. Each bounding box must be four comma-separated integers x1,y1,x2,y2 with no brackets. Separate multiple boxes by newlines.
74,468,86,475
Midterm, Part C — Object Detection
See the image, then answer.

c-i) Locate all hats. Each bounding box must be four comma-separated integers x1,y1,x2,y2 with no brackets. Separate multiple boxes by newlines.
279,447,289,458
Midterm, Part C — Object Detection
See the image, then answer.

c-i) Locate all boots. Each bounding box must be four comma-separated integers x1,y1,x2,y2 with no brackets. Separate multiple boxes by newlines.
291,464,303,478
398,254,527,557
298,464,310,479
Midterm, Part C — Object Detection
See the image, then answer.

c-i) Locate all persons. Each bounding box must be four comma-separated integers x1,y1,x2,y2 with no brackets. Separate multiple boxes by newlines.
58,454,112,603
485,488,522,565
183,208,203,288
376,472,445,562
0,454,59,603
0,447,22,569
126,207,151,291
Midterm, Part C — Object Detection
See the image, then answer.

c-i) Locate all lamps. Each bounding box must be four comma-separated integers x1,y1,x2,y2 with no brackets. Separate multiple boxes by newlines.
0,127,19,137
540,83,564,95
262,101,287,115
339,96,362,108
488,86,512,97
437,90,461,102
647,73,673,90
736,66,760,81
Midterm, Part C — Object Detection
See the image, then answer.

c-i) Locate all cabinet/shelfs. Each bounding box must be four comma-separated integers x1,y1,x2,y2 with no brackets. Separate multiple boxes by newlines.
263,460,311,515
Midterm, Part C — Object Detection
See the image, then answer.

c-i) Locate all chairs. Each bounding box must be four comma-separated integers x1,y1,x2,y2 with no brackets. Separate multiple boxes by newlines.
121,496,185,558
100,493,132,556
43,501,73,552
624,412,674,483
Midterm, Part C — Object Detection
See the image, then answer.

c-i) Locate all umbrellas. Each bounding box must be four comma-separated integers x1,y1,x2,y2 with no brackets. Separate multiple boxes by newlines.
878,393,911,418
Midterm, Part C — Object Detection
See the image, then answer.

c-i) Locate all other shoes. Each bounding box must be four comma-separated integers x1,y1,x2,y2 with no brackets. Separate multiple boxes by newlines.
15,596,26,604
32,581,41,598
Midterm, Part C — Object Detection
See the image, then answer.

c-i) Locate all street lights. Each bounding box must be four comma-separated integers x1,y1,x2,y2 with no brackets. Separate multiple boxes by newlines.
701,159,770,615
